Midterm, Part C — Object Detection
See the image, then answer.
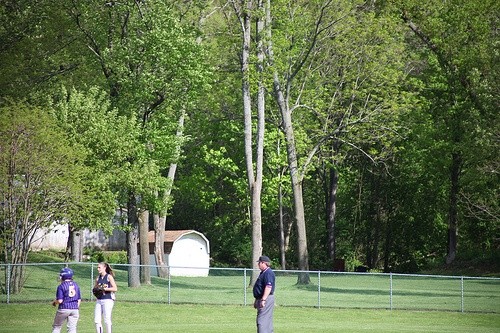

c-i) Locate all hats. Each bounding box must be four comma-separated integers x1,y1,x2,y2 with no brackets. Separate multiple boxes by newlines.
256,256,270,262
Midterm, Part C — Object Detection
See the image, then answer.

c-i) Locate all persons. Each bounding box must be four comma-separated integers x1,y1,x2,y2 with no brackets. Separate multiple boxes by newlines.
93,261,117,333
252,255,275,333
51,267,82,332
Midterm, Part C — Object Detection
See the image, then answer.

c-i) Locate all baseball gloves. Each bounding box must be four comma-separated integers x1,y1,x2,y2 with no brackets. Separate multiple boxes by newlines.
93,285,106,298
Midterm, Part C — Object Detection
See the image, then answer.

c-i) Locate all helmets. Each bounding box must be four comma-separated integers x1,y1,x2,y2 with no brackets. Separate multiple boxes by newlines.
59,267,74,281
